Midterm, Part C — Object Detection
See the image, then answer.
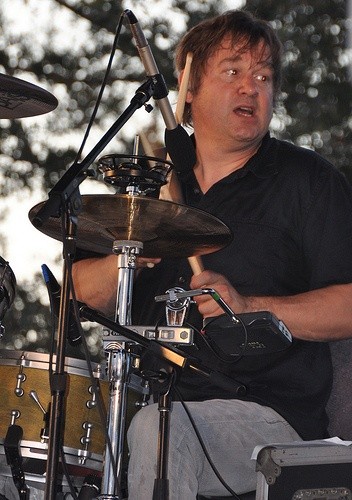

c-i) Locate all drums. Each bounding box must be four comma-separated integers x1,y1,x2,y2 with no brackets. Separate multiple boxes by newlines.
1,256,18,344
0,347,151,477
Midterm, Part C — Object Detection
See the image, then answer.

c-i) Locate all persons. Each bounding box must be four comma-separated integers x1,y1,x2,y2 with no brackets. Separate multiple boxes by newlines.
67,10,352,500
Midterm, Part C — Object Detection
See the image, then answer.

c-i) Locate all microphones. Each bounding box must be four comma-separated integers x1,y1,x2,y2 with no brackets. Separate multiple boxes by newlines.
125,11,197,171
41,264,83,347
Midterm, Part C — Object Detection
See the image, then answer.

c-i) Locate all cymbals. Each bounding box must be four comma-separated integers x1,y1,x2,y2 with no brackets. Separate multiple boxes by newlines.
0,69,60,120
26,193,233,259
